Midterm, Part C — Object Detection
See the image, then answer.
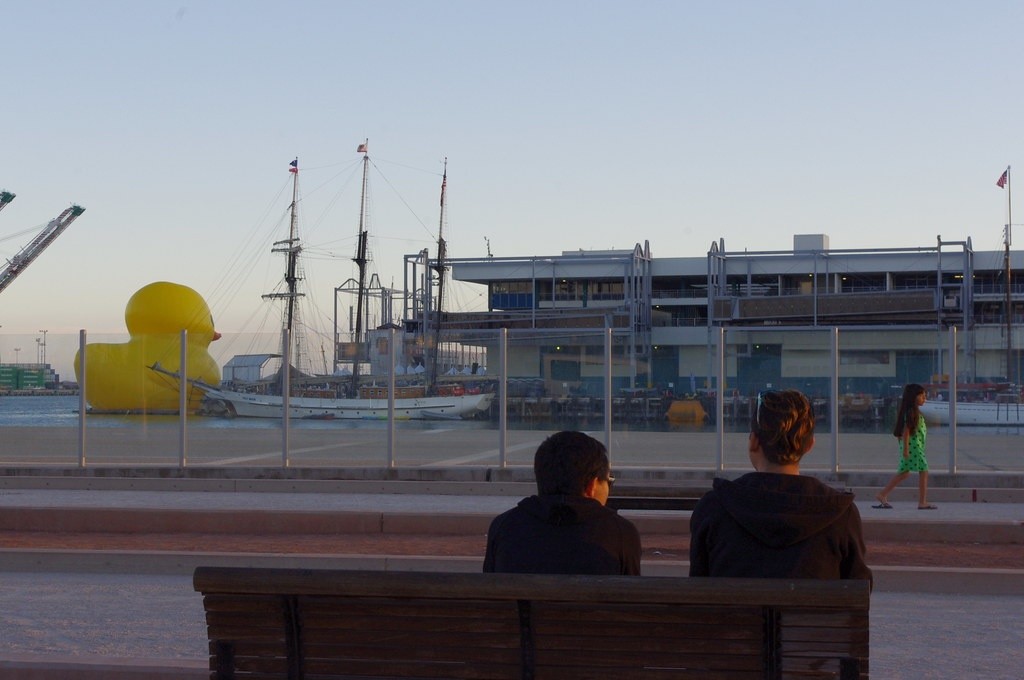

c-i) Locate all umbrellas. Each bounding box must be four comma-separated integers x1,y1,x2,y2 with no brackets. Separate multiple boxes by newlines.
460,367,472,375
447,368,459,376
476,368,486,375
384,364,425,376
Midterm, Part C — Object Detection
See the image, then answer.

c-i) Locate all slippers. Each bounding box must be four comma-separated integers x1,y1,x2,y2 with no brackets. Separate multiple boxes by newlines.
872,503,893,509
918,504,937,509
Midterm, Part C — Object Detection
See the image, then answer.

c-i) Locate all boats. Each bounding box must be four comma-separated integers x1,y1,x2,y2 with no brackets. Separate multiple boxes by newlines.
0,363,79,395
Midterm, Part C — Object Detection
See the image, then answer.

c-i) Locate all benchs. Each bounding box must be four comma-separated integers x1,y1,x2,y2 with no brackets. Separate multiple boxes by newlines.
192,567,870,680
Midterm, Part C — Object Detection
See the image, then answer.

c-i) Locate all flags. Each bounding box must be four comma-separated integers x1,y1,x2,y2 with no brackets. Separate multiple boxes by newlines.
997,170,1007,189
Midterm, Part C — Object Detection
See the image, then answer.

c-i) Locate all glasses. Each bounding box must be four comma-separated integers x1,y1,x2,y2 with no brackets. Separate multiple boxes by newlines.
757,389,815,430
605,474,615,488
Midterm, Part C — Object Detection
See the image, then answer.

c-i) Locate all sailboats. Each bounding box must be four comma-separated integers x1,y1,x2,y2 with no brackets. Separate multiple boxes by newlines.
239,137,492,396
897,222,1024,428
143,156,501,420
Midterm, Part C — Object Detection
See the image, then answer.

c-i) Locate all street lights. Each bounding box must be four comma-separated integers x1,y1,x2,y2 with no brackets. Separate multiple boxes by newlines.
36,338,40,363
40,343,46,363
14,347,21,364
40,330,49,363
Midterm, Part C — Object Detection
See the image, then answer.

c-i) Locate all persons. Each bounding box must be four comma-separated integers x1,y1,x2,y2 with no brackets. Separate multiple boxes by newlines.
484,431,642,578
688,389,873,592
569,380,581,394
872,384,937,509
